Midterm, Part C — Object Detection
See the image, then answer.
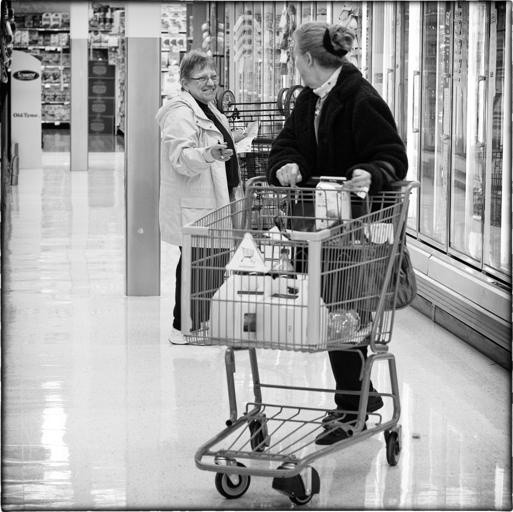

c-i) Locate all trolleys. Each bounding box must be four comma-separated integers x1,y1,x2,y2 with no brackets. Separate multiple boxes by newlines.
219,82,308,188
175,171,425,506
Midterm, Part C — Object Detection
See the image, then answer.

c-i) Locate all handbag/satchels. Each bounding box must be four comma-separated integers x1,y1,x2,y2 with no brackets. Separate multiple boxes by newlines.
346,159,417,313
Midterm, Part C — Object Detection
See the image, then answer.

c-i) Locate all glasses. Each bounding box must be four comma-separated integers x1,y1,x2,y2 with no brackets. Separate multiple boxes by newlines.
185,74,220,84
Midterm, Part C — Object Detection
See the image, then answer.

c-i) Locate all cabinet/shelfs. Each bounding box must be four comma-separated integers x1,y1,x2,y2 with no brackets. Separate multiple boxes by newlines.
1,1,194,137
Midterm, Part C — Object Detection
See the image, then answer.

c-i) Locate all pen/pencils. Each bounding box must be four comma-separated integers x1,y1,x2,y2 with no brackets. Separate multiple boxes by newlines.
217,140,224,160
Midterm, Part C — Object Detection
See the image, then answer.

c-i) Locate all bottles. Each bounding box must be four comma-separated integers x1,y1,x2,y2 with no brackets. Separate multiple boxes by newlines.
328,307,362,338
270,246,298,280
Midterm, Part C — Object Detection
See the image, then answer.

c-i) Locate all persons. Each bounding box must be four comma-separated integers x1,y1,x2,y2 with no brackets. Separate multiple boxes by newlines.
264,21,407,445
153,48,254,346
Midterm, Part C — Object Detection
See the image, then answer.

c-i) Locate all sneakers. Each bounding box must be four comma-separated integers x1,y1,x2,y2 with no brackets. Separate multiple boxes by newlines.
320,390,387,424
168,319,225,346
315,417,368,447
